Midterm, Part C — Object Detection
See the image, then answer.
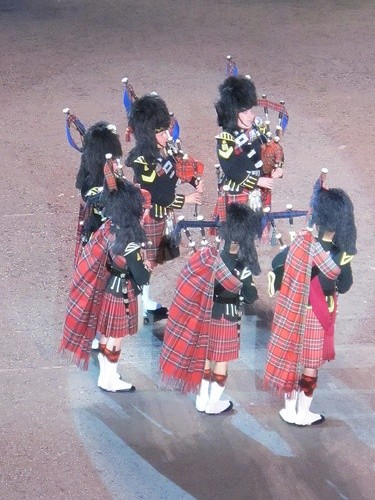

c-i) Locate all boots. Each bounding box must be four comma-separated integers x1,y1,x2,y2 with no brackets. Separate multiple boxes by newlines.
97,354,121,388
140,283,161,311
195,380,209,412
297,389,324,426
278,390,298,425
101,356,136,394
206,381,235,414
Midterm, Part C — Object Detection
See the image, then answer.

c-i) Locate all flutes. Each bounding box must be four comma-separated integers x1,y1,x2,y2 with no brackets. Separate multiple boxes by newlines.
63,106,125,180
121,75,201,190
177,213,221,253
265,168,329,249
104,151,152,251
226,55,286,173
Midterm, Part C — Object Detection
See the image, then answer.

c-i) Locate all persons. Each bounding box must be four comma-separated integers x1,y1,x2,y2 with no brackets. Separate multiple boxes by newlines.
64,121,150,284
60,175,152,393
209,76,289,249
263,188,357,426
159,201,262,415
121,92,206,270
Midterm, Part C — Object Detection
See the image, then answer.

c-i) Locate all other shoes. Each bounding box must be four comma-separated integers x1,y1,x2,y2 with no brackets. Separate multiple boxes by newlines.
90,338,100,350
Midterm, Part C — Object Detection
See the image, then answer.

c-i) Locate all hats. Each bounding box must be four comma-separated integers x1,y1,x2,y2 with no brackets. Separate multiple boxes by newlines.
217,185,263,276
63,107,124,189
99,152,146,253
312,167,359,256
215,54,258,128
122,78,171,153
